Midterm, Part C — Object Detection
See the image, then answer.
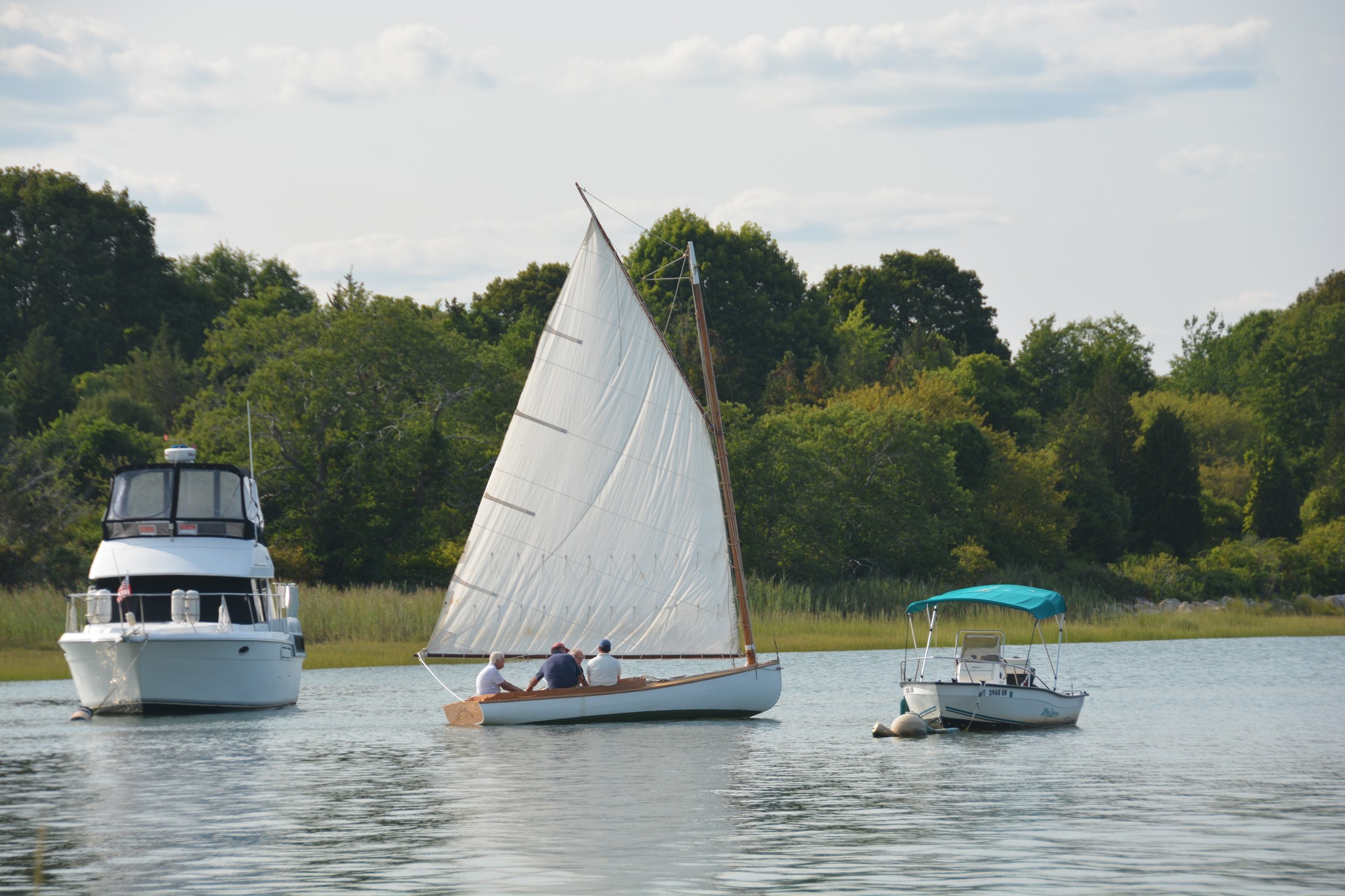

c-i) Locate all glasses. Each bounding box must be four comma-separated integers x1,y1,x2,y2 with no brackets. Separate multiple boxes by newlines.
562,648,567,652
572,654,584,661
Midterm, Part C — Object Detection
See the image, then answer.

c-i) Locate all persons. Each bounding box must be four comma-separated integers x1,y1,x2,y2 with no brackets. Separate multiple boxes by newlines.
587,639,621,686
526,643,589,691
475,651,523,696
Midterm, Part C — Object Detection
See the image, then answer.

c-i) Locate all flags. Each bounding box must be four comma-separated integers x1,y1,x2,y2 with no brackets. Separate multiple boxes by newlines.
117,578,131,603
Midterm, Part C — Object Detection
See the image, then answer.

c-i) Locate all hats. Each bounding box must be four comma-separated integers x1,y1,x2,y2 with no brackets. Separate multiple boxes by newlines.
551,643,570,653
599,639,611,649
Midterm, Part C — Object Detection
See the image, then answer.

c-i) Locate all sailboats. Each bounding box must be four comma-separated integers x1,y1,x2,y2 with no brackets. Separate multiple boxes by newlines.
409,179,785,730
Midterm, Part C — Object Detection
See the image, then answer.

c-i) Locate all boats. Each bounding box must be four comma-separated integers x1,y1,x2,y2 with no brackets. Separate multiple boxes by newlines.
54,441,307,711
899,584,1090,732
